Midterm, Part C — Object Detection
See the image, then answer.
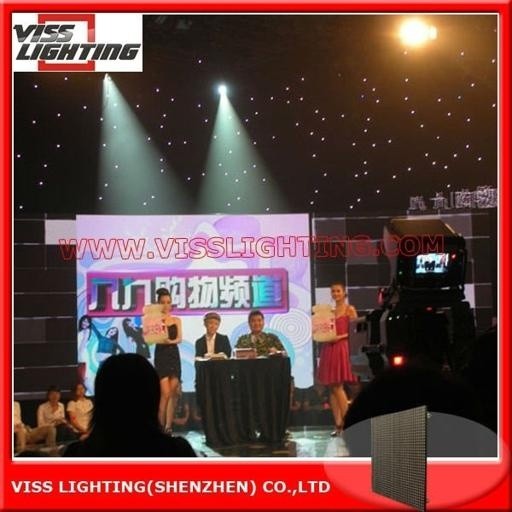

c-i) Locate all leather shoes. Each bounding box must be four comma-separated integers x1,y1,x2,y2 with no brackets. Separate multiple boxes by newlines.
330,426,343,436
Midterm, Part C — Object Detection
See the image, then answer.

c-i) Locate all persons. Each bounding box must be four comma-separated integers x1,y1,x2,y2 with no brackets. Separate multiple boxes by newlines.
62,352,198,457
191,376,202,428
124,318,151,360
90,322,125,367
195,312,232,359
417,254,446,271
66,382,93,432
154,287,182,435
319,279,359,437
289,371,362,426
36,386,89,442
77,315,92,378
14,401,58,455
236,310,287,357
173,380,190,432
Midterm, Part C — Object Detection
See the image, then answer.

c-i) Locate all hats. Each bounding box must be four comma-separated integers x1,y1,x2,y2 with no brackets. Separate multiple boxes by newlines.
204,312,221,322
